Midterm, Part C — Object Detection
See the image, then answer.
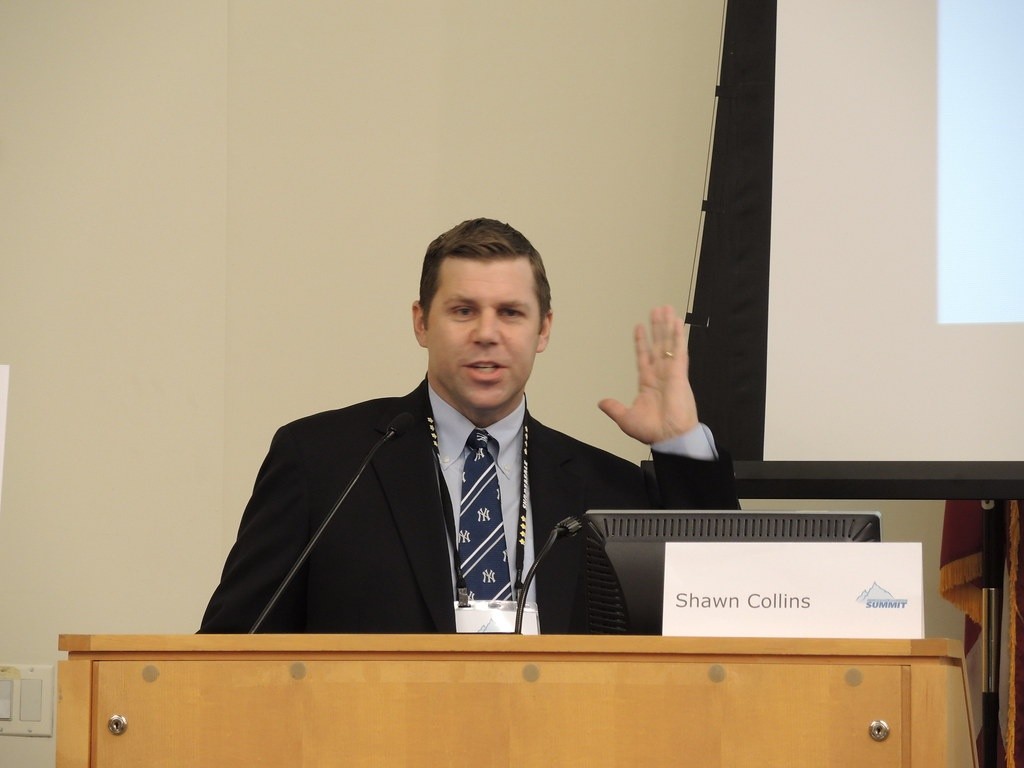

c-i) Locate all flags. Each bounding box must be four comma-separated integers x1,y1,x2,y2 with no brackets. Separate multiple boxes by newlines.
939,497,1016,666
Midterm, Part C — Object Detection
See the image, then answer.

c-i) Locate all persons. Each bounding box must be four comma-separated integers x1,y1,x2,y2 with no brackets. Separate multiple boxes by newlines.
192,218,738,632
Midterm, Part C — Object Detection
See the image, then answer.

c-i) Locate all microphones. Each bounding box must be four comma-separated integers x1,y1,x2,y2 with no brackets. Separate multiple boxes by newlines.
516,515,581,635
249,412,417,634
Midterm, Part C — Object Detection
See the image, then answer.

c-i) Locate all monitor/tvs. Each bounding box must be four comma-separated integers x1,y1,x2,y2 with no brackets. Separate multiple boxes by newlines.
583,508,880,634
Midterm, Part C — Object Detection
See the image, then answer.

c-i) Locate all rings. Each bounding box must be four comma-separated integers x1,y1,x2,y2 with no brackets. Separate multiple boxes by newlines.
663,351,672,358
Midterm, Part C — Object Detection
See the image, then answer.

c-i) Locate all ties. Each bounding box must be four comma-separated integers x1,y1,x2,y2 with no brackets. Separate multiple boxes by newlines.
455,427,512,600
